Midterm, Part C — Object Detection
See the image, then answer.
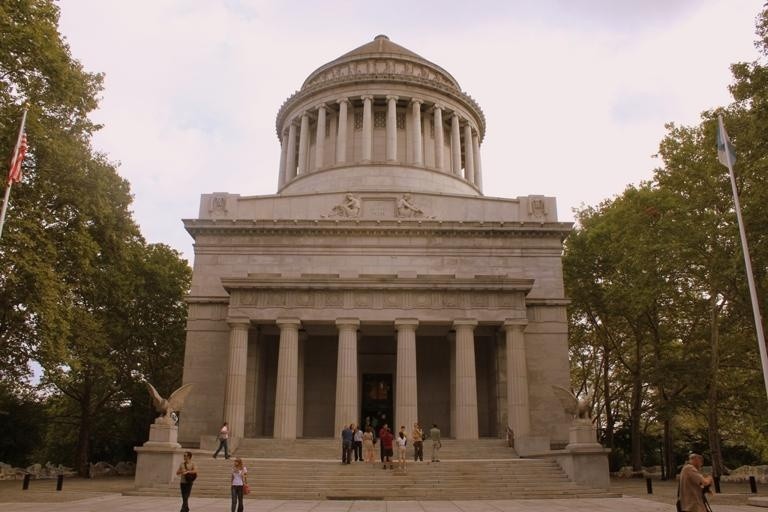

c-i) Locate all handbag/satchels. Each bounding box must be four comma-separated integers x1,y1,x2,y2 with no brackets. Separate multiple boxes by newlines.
185,471,197,482
242,483,250,496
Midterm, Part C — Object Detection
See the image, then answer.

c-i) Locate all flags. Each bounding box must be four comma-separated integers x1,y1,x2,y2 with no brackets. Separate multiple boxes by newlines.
8,127,30,188
713,120,738,173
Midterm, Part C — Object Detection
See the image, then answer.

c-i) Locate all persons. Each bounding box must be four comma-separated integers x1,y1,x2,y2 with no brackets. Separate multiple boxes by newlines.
676,453,714,512
212,422,231,459
396,192,433,219
229,457,250,511
176,449,197,511
339,413,443,472
323,192,361,219
